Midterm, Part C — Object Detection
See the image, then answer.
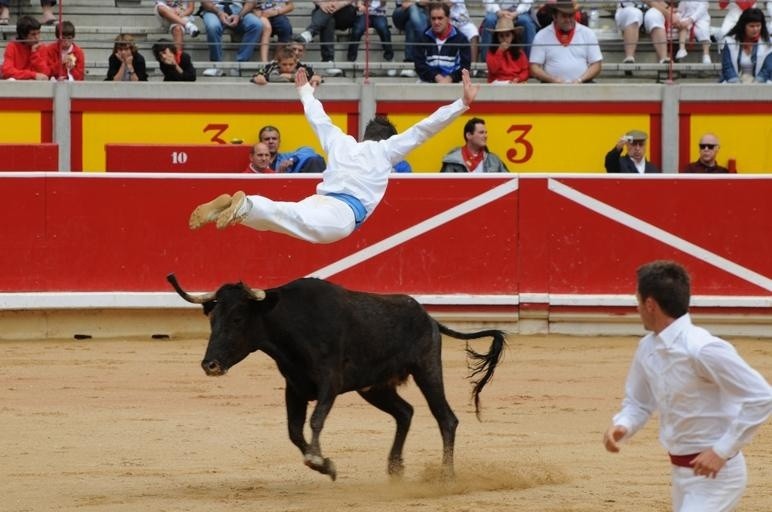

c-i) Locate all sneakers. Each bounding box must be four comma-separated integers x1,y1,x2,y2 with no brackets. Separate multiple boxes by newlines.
659,58,673,64
400,68,417,77
385,59,397,76
296,31,313,45
623,56,635,62
39,15,56,25
675,49,688,59
216,190,249,230
702,55,711,64
202,67,223,77
324,67,343,77
230,69,255,76
0,16,9,25
185,23,200,37
189,194,231,230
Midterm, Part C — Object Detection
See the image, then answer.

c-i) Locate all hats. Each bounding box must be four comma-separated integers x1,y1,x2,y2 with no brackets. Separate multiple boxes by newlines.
486,17,524,35
545,0,583,14
625,130,647,142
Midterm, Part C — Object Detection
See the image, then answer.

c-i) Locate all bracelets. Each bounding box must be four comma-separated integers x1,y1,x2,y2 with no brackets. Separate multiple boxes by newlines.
127,69,135,75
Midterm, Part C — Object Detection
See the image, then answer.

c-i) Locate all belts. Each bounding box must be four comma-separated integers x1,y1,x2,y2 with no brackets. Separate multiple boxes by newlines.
667,453,702,468
324,192,368,229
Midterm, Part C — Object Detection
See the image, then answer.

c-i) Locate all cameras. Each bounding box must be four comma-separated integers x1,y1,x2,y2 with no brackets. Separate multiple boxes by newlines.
623,136,633,143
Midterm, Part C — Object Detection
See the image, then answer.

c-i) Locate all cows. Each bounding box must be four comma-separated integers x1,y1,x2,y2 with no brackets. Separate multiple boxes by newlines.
166,270,510,485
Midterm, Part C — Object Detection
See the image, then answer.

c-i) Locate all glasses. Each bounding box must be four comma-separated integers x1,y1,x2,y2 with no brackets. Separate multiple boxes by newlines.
431,16,444,20
698,143,719,150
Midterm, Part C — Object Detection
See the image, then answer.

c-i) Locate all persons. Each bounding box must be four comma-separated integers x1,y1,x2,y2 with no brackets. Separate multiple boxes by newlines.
529,0,604,84
614,0,681,78
200,0,263,78
294,0,356,77
480,0,537,75
257,125,300,174
0,0,58,24
718,8,772,84
152,38,197,82
484,18,529,84
254,0,294,63
719,0,758,39
676,0,713,65
48,20,85,82
189,66,481,246
347,0,398,76
243,141,277,173
415,3,471,86
443,0,481,79
268,48,310,81
154,0,199,50
604,130,659,174
601,259,772,512
441,117,509,173
683,132,730,174
1,15,51,82
392,0,429,63
103,33,149,81
250,34,324,86
